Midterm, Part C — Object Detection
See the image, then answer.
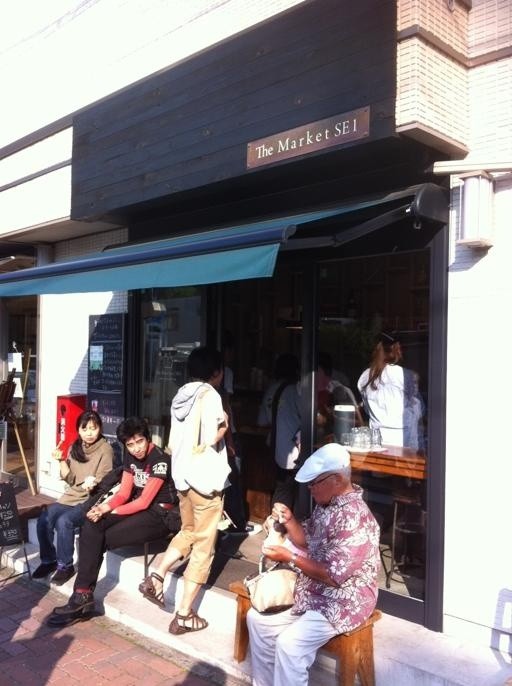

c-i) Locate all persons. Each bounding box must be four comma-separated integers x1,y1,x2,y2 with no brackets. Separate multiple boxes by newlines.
245,442,381,685
31,411,113,586
221,331,426,534
138,346,233,634
46,416,182,627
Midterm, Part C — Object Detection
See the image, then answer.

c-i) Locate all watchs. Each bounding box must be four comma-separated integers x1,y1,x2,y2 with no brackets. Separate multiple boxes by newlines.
287,552,298,568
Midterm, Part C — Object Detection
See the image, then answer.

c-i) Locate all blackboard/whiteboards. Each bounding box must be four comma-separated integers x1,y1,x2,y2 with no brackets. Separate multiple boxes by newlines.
87,312,126,465
0,483,22,546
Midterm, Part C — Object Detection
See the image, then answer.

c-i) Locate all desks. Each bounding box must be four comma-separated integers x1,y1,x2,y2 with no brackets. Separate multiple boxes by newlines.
312,432,430,483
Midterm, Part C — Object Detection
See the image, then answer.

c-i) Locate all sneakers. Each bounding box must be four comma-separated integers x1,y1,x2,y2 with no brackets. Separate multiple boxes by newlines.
31,561,58,578
51,567,76,585
230,522,263,537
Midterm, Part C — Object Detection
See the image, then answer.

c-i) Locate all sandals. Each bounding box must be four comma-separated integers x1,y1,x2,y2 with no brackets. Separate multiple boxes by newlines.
138,573,165,608
169,610,207,634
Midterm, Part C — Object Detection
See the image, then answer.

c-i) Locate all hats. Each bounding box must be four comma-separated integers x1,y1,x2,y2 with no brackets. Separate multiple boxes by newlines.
295,443,350,483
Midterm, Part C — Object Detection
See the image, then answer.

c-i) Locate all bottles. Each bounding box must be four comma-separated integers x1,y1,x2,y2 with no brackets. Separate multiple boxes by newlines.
334,404,355,446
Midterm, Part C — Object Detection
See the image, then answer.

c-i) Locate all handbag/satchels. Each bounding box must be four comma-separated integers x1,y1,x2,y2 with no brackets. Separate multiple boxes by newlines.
244,567,300,613
160,504,181,531
184,445,232,497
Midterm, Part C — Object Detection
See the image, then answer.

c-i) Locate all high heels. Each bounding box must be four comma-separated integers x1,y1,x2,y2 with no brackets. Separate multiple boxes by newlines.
52,592,95,616
48,613,88,627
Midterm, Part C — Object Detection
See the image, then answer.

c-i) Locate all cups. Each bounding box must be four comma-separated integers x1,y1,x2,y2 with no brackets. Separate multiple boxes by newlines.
91,400,99,413
341,426,383,447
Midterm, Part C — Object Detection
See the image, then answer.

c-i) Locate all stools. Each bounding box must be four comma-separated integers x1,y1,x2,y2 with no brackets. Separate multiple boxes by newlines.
385,490,429,590
224,574,389,686
139,531,184,584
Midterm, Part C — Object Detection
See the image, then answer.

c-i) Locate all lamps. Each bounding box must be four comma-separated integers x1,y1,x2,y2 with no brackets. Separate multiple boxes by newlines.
455,165,499,254
